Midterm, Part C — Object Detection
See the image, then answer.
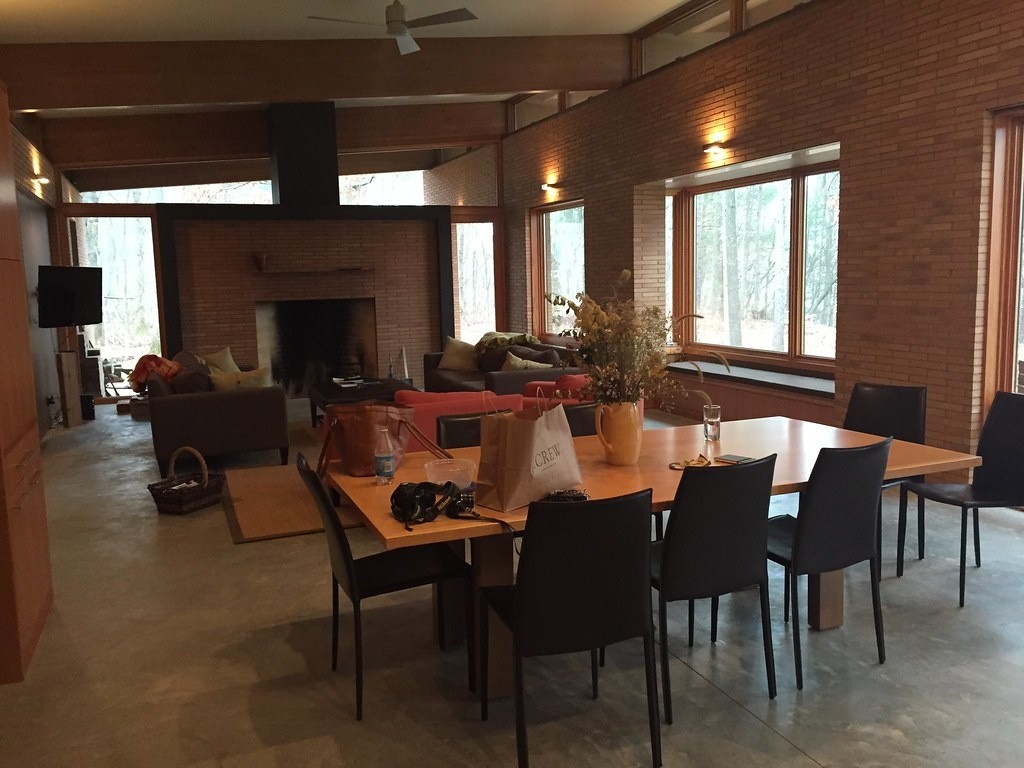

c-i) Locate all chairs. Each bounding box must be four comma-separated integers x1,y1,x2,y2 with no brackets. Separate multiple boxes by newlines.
564,401,663,542
481,487,663,768
897,390,1024,609
437,409,526,539
295,451,479,721
799,382,927,585
599,453,778,726
709,435,894,694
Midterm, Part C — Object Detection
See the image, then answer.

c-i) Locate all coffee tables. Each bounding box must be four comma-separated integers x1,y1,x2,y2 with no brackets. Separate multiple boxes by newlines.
309,377,421,429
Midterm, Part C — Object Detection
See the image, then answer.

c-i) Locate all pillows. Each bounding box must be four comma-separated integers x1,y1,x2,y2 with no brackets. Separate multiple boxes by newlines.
202,346,240,372
437,335,482,371
500,352,554,371
208,366,275,391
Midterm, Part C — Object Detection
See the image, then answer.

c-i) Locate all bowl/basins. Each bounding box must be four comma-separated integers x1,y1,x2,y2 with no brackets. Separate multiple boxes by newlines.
424,459,475,489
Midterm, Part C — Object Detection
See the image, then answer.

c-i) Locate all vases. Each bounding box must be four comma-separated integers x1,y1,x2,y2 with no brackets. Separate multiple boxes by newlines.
596,398,641,466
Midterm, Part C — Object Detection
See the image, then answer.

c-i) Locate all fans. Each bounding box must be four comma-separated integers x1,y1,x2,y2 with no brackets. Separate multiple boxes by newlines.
306,0,478,56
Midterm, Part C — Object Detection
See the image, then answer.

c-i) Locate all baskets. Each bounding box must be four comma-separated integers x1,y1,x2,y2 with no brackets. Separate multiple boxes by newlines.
148,446,226,515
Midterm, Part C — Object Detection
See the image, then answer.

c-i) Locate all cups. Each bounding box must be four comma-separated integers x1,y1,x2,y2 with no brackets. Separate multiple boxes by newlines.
703,404,721,442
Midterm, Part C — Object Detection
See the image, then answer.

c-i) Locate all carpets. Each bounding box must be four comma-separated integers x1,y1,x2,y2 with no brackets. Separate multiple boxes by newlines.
216,464,365,545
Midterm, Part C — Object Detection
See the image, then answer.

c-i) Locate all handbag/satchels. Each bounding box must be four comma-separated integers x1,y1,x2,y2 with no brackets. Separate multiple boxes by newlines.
474,387,583,512
316,403,453,480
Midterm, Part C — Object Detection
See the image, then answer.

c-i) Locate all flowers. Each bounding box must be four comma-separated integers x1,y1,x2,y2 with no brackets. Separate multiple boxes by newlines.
542,269,731,418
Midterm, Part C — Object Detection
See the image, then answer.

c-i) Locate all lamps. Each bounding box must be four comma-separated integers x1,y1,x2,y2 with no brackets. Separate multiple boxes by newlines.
539,183,561,191
703,141,725,155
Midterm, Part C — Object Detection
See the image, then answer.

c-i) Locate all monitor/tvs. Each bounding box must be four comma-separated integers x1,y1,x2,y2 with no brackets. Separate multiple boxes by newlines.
37,266,102,329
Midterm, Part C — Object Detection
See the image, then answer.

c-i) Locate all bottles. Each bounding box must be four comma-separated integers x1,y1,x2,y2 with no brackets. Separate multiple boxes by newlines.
374,429,394,484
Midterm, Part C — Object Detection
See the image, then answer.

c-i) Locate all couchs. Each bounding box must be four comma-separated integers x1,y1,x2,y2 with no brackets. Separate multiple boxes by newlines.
147,365,290,476
323,383,645,460
423,343,590,398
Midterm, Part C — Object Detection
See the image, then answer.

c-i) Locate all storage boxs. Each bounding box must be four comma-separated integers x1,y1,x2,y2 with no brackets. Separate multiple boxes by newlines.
422,459,476,490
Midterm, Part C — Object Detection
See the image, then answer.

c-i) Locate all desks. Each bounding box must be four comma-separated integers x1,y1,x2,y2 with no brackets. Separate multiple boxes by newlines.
327,417,983,698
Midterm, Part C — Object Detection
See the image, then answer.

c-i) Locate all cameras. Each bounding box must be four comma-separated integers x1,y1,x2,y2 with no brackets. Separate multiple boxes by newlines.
447,493,474,517
391,487,436,523
549,489,588,502
